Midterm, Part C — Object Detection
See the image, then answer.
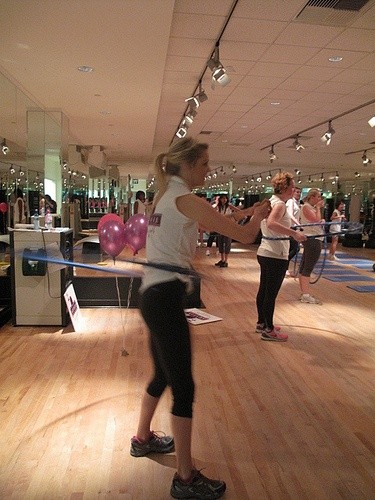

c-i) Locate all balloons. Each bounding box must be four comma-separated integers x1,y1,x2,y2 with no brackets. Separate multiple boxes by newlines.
98,212,127,261
126,212,150,256
0,202,7,214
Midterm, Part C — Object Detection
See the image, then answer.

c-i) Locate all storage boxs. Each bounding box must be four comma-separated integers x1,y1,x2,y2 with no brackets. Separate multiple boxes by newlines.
81,242,102,264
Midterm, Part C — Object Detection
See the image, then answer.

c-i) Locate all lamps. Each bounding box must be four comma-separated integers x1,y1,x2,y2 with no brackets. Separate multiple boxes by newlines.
335,171,339,179
9,164,16,175
292,134,309,154
362,150,372,167
182,108,200,125
206,41,236,91
36,172,40,180
190,165,273,194
19,166,25,176
297,177,302,184
184,79,209,110
0,138,10,155
175,119,189,139
268,144,280,164
320,173,326,182
320,120,337,145
354,172,361,178
308,176,313,183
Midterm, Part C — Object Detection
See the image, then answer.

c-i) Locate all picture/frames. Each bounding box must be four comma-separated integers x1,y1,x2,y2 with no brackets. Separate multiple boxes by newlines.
132,179,138,184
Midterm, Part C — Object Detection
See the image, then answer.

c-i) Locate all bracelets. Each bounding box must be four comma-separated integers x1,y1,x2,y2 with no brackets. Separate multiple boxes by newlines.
232,213,238,222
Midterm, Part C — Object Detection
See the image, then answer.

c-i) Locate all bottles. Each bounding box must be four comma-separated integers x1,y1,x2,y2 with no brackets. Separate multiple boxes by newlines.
45,211,52,229
34,211,40,229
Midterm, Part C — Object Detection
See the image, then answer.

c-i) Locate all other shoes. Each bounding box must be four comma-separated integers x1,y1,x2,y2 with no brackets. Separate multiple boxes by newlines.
220,262,228,267
215,260,225,266
216,251,219,255
206,251,210,256
329,255,340,261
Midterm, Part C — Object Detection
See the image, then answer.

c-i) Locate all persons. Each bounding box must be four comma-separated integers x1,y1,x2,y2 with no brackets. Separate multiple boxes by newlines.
284,186,302,276
39,194,57,215
134,191,154,218
194,194,244,267
298,189,325,303
13,189,26,224
256,172,306,342
327,200,347,260
131,137,273,500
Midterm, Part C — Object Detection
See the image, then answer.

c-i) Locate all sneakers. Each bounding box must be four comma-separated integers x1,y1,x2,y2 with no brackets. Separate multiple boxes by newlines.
298,294,320,304
170,467,226,500
130,430,176,457
255,321,281,334
260,329,288,342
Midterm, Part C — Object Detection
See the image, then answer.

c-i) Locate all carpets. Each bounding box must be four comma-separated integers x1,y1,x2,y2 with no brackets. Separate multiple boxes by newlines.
292,248,375,294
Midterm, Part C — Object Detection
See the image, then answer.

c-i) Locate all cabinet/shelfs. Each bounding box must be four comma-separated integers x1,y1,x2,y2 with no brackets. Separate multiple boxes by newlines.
7,225,73,328
